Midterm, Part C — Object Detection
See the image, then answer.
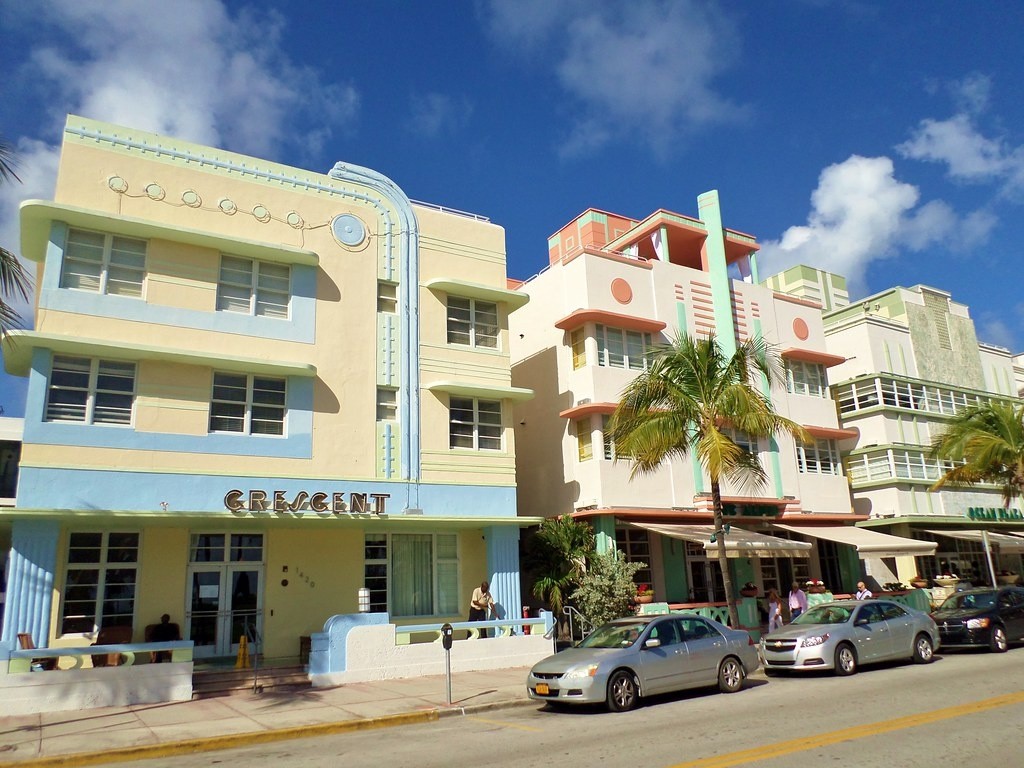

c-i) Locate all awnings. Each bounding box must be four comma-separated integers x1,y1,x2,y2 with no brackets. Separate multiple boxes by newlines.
916,527,1024,554
773,523,936,559
614,517,811,558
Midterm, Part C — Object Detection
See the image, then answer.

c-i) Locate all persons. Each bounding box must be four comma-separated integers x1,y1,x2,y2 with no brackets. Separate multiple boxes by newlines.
789,582,806,621
806,580,834,609
151,614,178,661
467,581,500,639
768,589,782,632
856,582,874,619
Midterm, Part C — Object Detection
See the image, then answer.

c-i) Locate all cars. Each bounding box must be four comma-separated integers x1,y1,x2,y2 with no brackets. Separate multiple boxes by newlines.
932,587,1024,653
526,615,761,715
758,599,943,676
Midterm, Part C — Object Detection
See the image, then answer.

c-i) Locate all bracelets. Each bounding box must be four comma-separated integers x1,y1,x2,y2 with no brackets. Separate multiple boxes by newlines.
777,613,780,615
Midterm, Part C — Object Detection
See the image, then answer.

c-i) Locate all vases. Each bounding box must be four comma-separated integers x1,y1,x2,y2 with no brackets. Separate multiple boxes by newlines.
739,590,759,597
996,575,1020,584
808,585,826,593
631,596,653,603
933,578,961,587
911,581,927,588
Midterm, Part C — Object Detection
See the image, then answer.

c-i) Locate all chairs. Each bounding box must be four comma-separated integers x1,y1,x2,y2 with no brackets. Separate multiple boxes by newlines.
89,627,132,668
145,621,184,662
17,633,62,673
885,607,898,619
694,625,709,639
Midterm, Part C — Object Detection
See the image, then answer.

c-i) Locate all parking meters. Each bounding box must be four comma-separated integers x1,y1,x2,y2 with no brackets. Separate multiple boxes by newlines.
441,623,454,708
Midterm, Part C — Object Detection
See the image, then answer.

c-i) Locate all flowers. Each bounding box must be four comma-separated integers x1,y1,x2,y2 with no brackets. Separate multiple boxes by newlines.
806,577,824,587
909,576,927,582
636,584,656,596
936,573,959,580
997,569,1017,576
882,582,908,591
741,582,760,591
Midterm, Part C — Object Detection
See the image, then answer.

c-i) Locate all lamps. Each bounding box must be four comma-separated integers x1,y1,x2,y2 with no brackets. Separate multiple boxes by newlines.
519,333,524,337
520,418,526,425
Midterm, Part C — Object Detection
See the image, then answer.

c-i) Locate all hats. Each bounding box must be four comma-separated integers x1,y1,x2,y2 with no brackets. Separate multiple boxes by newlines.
805,581,814,586
792,582,798,586
816,580,824,586
